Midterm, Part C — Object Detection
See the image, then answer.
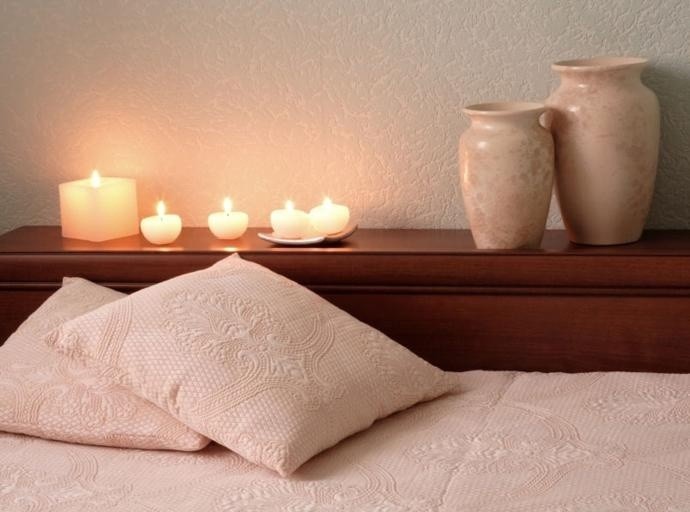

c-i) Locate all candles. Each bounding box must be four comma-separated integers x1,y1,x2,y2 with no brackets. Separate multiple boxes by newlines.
139,200,182,245
270,200,310,239
309,197,350,235
59,169,140,242
207,196,249,240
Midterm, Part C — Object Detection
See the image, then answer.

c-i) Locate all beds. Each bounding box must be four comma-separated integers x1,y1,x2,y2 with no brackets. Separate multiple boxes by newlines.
1,225,690,512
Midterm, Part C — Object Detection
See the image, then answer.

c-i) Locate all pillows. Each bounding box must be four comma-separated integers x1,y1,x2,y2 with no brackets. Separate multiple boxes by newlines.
0,276,212,451
40,252,471,478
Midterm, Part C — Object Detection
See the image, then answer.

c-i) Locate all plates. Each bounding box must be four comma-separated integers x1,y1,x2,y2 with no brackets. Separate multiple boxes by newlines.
256,224,359,245
